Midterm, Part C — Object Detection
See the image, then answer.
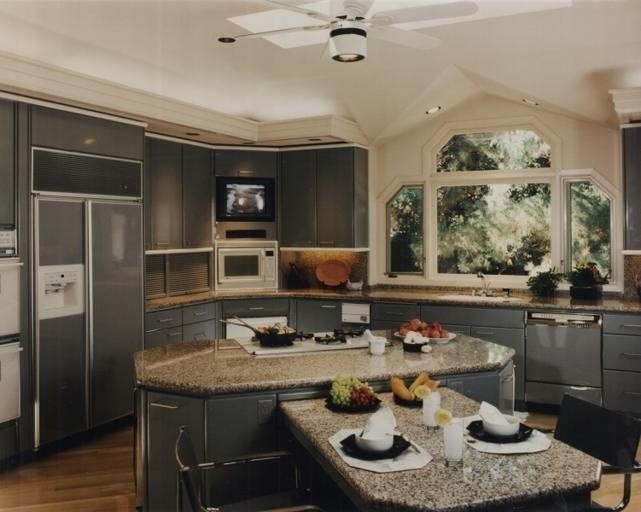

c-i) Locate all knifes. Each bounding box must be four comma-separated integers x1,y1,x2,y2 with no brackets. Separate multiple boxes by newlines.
287,262,305,274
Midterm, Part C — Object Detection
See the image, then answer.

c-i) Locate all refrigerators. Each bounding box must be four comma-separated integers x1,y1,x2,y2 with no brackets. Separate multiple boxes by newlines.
27,186,147,442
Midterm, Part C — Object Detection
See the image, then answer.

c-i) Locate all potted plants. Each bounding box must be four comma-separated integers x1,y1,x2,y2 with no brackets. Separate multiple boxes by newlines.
526,271,563,304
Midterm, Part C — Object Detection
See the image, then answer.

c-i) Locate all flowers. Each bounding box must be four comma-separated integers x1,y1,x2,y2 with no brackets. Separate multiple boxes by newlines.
564,262,609,288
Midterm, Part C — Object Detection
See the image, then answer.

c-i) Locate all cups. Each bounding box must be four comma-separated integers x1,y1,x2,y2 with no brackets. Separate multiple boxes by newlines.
422,385,443,431
439,417,465,467
368,337,388,356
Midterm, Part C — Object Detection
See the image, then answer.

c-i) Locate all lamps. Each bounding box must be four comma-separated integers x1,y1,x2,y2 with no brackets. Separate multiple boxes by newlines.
329,28,367,62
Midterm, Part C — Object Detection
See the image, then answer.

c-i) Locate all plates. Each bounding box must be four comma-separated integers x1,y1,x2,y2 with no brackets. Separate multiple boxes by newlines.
392,328,456,344
466,420,533,443
336,431,412,460
315,258,352,288
393,392,423,405
325,396,381,413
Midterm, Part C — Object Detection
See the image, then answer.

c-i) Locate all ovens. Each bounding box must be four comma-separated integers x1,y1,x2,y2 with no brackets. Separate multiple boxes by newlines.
521,301,603,411
1,248,25,426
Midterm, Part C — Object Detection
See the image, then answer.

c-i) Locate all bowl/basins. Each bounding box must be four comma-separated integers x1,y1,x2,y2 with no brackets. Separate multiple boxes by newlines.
402,338,427,352
354,426,394,451
482,413,520,437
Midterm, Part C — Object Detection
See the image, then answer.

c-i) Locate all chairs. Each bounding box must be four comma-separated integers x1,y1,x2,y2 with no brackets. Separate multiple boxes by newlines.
173,426,320,512
553,394,641,512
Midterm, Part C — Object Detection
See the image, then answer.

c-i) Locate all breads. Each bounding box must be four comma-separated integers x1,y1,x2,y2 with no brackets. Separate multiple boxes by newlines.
390,370,438,402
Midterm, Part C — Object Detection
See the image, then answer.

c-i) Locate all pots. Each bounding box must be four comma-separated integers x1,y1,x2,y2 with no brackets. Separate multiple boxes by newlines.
219,315,297,346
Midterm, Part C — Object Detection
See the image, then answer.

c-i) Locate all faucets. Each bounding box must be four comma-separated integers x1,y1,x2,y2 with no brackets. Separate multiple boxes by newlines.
477,272,487,293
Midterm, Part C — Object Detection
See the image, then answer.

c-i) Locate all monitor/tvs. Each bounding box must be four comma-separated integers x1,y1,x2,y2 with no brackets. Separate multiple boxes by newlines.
214,175,276,222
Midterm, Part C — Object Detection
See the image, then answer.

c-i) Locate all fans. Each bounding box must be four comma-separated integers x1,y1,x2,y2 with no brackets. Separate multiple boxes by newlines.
235,0,478,50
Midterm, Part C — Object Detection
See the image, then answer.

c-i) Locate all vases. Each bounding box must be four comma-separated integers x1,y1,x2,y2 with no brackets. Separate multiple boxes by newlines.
570,286,602,299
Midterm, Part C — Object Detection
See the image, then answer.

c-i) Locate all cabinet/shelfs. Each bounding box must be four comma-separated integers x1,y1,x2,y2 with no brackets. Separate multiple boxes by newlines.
441,323,524,413
297,299,342,333
282,146,368,248
602,313,641,413
214,150,277,178
148,390,204,512
223,299,289,317
143,136,210,250
372,303,421,322
145,302,215,348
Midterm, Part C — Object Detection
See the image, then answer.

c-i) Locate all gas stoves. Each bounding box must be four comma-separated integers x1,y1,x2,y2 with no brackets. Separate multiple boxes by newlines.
231,325,392,356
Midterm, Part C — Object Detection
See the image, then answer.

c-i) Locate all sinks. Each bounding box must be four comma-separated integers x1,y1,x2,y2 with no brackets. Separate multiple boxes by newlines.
457,292,515,297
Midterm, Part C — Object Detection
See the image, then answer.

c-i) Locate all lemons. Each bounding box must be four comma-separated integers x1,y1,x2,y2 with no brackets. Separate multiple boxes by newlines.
434,409,451,428
330,373,378,408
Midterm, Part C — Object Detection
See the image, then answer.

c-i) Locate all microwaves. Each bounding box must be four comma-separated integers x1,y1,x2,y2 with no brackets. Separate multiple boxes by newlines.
213,240,278,291
